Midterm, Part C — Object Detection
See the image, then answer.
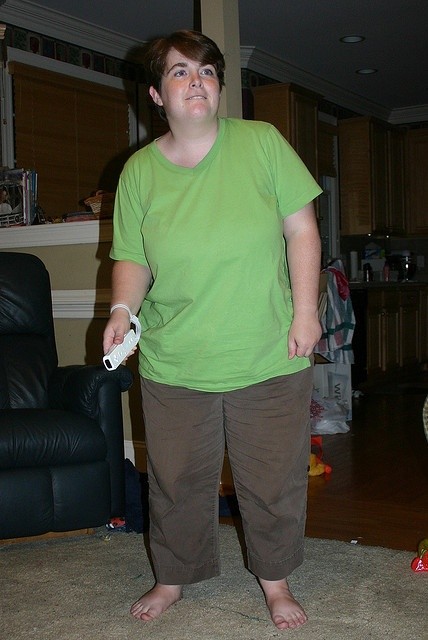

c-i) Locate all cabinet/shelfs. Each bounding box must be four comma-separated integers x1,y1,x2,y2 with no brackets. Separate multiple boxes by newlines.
255,83,321,223
405,122,428,234
338,114,404,236
352,284,426,390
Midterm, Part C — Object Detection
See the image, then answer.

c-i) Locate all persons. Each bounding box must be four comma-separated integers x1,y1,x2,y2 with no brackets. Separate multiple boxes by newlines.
103,30,323,630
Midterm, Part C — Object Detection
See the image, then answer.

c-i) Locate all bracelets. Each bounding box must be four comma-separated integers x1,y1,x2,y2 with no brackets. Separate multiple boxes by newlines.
110,304,142,345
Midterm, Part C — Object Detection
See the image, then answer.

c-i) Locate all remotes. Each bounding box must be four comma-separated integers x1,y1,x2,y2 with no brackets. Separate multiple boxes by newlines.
100,311,142,375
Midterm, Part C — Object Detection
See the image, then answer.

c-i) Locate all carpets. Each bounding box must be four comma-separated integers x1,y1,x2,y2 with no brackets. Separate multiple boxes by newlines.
0,523,428,639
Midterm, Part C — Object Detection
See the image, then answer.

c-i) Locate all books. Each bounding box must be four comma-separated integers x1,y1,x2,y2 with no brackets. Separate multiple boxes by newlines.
0,168,38,226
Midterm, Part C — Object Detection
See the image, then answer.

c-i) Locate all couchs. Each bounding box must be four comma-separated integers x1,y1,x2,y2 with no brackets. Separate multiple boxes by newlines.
0,250,134,539
